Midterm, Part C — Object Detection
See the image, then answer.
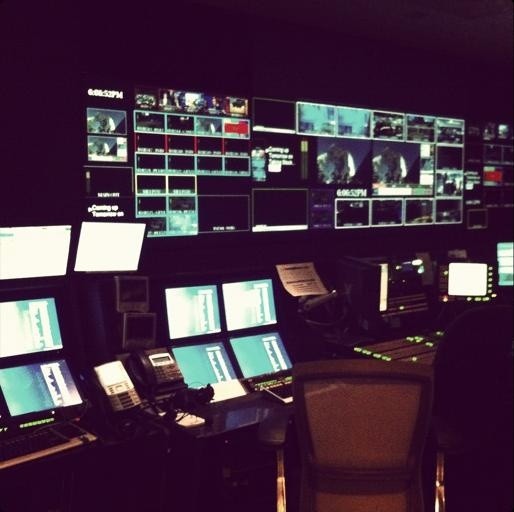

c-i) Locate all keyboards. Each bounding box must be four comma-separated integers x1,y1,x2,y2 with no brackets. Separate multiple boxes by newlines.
261,379,338,404
0,422,98,470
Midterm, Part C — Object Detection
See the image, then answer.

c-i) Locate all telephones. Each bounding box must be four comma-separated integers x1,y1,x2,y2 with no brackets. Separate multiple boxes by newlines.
128,346,185,394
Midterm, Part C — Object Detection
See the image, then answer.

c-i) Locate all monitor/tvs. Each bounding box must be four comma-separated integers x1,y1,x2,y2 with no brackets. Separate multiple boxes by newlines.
465,117,513,230
251,97,464,234
114,276,150,312
0,357,88,419
448,262,497,305
81,83,250,237
496,242,514,287
162,283,222,340
227,331,294,379
221,278,279,331
0,296,65,358
73,221,147,274
122,313,156,349
0,224,73,281
168,341,239,389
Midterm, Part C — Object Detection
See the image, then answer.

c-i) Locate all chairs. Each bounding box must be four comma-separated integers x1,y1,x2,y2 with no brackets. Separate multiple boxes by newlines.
277,358,446,512
